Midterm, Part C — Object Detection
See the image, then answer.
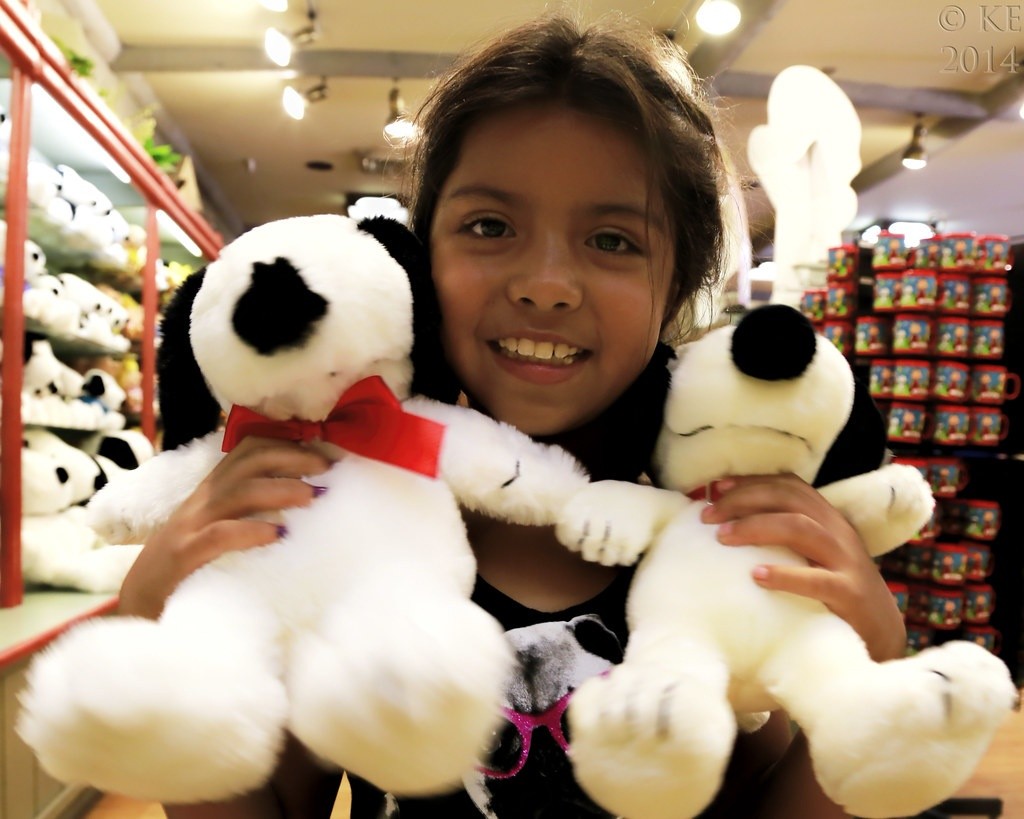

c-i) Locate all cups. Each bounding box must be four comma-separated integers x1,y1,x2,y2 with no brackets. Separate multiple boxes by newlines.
799,225,1024,658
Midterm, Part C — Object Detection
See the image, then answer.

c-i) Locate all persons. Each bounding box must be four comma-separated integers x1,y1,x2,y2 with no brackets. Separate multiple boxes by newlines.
108,13,912,819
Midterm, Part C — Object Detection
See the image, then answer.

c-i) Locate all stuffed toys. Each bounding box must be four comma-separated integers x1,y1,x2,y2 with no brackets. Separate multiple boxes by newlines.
25,201,587,802
550,304,1013,819
2,78,213,616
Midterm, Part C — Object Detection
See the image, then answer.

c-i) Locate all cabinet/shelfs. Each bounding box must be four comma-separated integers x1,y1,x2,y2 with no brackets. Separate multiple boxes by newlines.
2,0,229,817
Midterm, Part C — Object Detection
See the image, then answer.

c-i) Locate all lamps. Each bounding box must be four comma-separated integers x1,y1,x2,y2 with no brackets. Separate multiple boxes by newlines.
282,83,322,119
262,28,311,65
383,88,415,147
901,116,933,168
